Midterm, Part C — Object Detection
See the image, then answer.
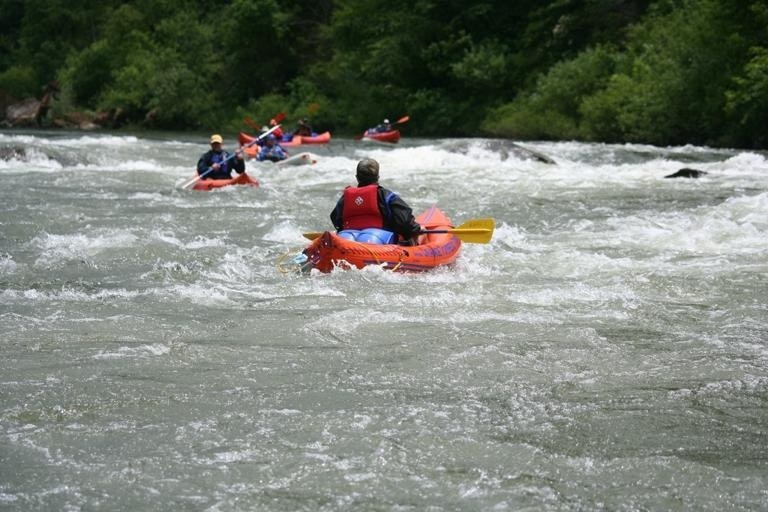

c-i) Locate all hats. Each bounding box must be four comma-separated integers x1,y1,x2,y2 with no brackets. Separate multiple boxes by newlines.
383,118,390,124
260,125,276,140
357,158,379,179
209,133,223,144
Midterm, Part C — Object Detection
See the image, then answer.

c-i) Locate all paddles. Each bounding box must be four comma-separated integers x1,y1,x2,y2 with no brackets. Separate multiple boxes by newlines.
303,216,494,244
177,124,281,191
243,117,262,133
390,116,409,127
274,112,286,123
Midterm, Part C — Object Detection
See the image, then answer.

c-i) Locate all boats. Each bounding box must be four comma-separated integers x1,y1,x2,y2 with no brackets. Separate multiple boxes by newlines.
272,152,315,165
299,202,461,276
190,171,261,190
290,133,331,142
233,143,261,161
362,128,399,142
238,130,302,148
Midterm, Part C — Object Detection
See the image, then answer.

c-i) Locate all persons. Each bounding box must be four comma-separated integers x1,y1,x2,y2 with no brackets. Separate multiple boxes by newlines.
330,158,427,244
255,119,288,162
295,117,312,136
376,118,392,132
197,134,244,180
